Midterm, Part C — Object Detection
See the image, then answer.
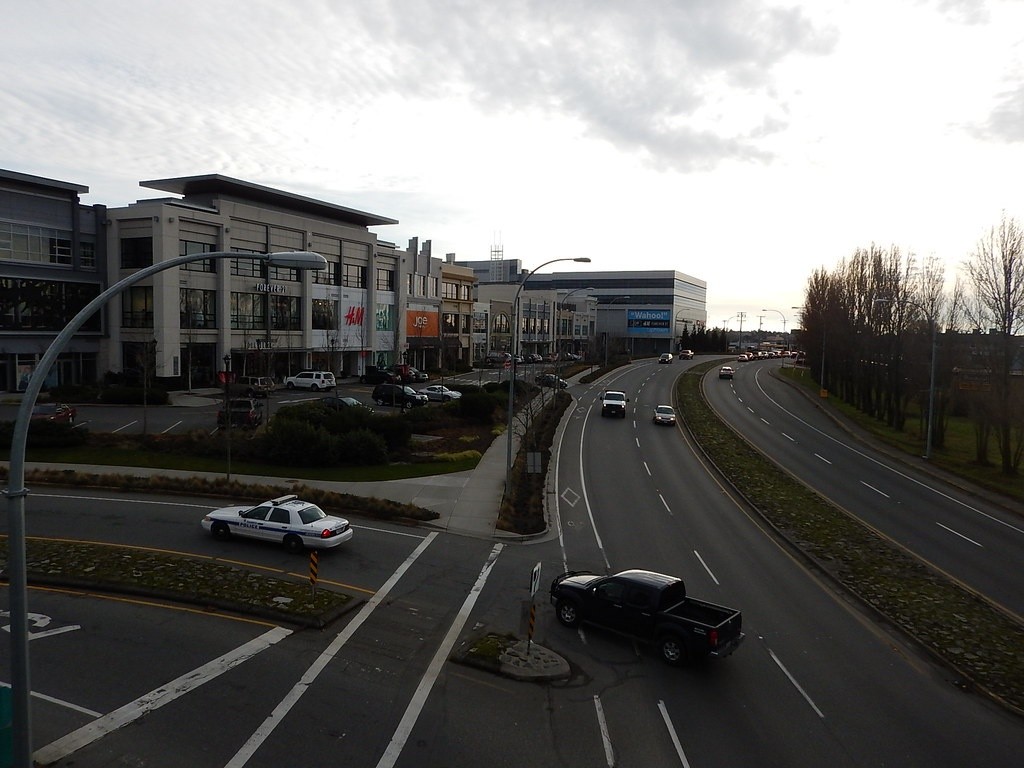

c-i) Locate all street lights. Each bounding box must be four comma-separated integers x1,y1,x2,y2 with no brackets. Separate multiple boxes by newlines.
762,309,785,369
9,249,330,768
557,286,595,396
791,306,826,398
506,257,593,500
875,298,935,458
675,308,691,337
222,353,234,481
605,296,630,369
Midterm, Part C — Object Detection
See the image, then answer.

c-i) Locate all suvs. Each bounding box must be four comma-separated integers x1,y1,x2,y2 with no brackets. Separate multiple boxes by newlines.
238,374,276,398
284,369,337,393
372,383,429,409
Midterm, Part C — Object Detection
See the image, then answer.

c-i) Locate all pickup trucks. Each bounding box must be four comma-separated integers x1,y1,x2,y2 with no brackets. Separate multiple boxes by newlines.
549,565,749,671
678,349,695,360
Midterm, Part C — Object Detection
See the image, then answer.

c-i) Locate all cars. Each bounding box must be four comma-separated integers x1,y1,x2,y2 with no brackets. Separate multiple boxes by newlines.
418,384,462,404
217,396,264,433
652,404,677,427
199,494,354,557
737,345,806,366
718,365,735,379
371,363,429,385
600,389,630,418
31,401,77,425
484,350,582,369
658,352,674,364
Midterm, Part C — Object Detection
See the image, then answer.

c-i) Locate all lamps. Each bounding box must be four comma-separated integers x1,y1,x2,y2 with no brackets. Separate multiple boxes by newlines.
107,219,112,225
154,216,160,222
374,253,377,257
227,228,230,232
170,218,174,222
403,259,406,262
439,266,442,270
308,242,312,247
102,220,107,224
